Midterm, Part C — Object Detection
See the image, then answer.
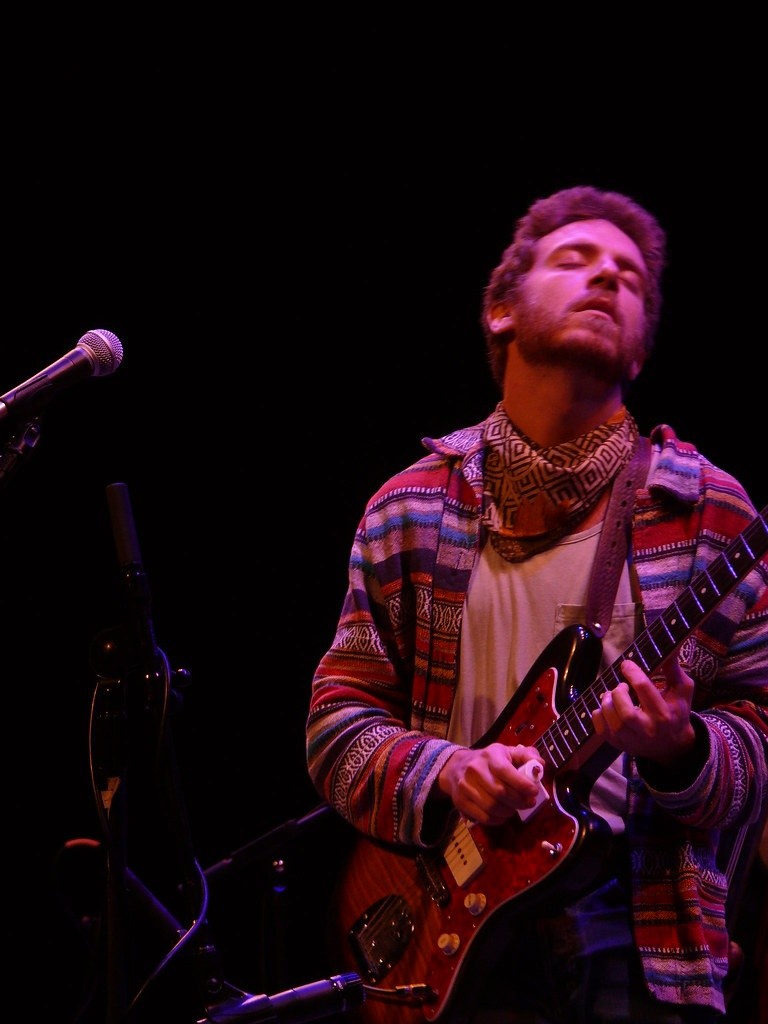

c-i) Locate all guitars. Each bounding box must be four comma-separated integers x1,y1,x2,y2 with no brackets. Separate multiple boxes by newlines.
336,502,767,1024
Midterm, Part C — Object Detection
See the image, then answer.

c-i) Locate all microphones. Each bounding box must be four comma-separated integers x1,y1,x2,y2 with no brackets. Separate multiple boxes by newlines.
103,482,162,676
0,327,123,423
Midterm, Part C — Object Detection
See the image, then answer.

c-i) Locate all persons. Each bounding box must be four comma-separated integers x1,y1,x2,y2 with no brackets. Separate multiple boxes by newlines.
305,186,766,1023
